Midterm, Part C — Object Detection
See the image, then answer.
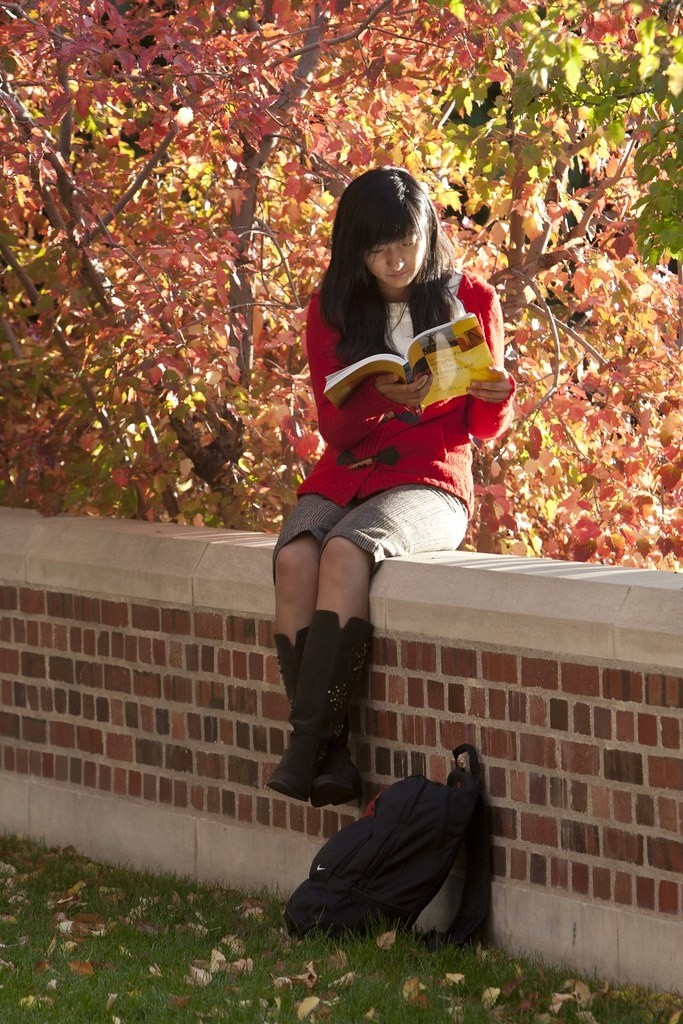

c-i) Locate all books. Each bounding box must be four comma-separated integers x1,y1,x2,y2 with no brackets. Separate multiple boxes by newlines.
322,313,501,417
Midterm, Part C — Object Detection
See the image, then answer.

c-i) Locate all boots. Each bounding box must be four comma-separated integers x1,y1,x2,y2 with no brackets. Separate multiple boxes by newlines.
267,609,375,802
273,625,363,808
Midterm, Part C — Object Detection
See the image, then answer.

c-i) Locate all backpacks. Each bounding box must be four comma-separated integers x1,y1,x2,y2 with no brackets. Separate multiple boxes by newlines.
284,743,491,952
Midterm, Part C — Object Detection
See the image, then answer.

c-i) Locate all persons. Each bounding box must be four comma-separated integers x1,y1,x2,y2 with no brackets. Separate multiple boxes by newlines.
268,166,516,808
